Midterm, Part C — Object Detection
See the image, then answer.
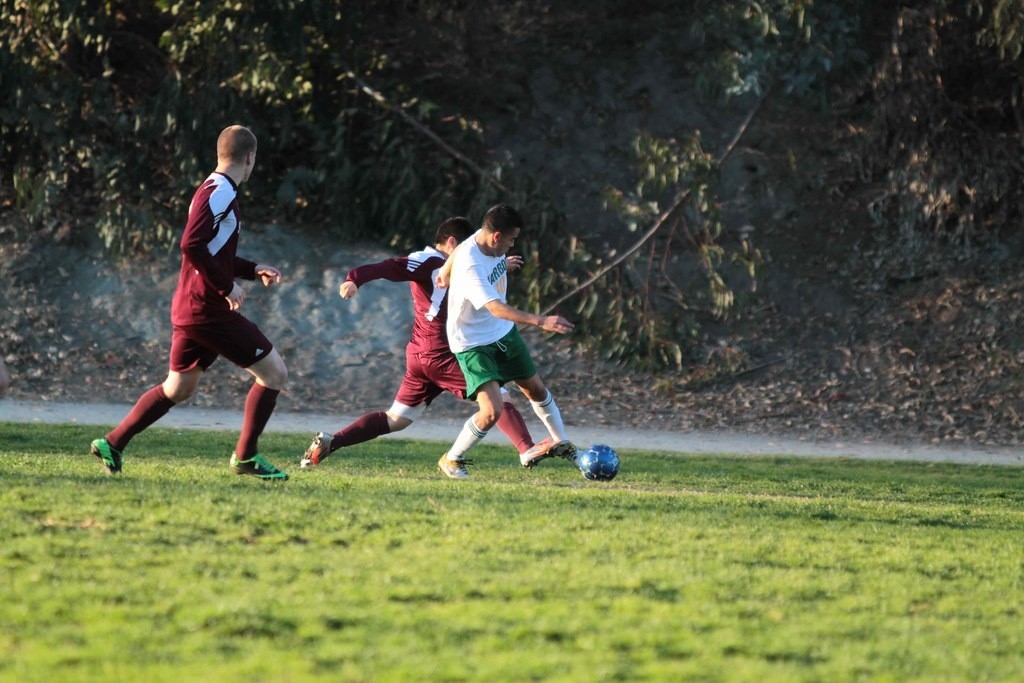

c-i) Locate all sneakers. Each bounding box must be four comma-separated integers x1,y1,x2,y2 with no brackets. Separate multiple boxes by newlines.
90,434,126,480
229,450,290,480
565,445,585,471
437,451,470,480
300,430,334,469
518,437,571,471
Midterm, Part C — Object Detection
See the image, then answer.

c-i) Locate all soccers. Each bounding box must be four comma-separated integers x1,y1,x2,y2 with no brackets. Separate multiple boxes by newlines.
579,444,621,481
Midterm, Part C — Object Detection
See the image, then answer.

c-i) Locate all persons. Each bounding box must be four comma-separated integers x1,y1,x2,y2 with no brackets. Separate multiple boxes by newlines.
433,204,588,482
299,216,573,474
90,124,293,482
